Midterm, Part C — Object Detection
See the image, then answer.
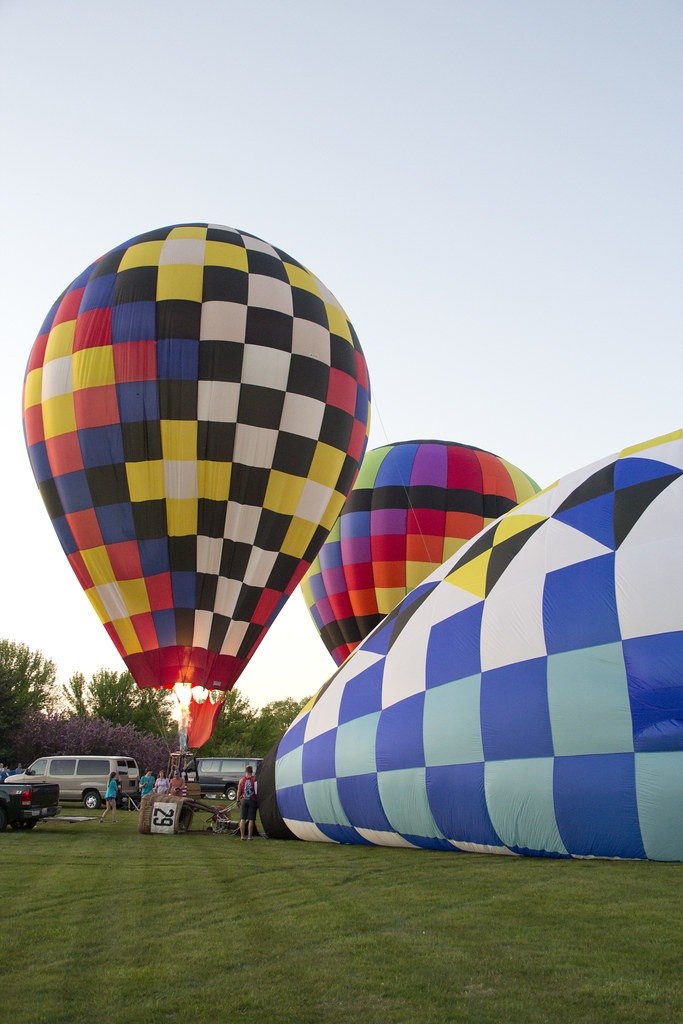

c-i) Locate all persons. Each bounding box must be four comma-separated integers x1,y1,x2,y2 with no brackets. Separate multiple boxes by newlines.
138,770,156,797
167,770,185,797
0,763,14,783
99,772,120,823
14,763,24,774
236,766,259,841
154,770,170,794
169,758,179,782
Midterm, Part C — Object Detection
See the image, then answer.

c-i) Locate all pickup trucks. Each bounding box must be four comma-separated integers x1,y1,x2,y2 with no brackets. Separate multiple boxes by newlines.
0,781,61,831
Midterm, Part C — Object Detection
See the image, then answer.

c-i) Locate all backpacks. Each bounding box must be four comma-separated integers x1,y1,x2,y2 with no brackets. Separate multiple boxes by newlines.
242,776,255,800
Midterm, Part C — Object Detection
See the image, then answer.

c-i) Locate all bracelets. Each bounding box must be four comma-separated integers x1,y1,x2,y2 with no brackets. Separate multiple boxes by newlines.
237,800,240,802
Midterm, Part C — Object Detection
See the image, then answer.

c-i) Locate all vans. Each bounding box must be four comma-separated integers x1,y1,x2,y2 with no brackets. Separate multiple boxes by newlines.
183,757,264,801
4,755,140,809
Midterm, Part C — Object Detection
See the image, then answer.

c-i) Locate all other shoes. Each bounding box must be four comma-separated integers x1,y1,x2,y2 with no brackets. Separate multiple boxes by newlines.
112,820,119,822
248,837,253,840
99,819,103,823
241,837,246,840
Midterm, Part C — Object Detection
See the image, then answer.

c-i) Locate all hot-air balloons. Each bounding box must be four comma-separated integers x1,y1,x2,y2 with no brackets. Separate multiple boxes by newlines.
21,223,373,797
297,440,543,668
136,427,682,865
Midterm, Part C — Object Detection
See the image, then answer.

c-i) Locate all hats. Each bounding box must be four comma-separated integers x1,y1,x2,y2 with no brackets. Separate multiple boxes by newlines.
174,770,179,775
